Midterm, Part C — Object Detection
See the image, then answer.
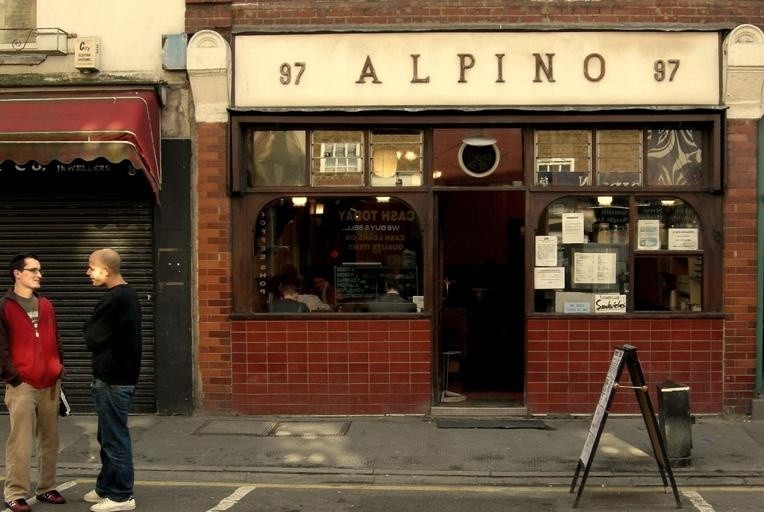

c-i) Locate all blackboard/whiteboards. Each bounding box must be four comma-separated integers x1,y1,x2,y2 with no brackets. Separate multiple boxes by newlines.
332,264,420,307
577,345,626,472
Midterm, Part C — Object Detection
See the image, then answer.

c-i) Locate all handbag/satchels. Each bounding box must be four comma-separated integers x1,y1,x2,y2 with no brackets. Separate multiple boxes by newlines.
60,385,72,417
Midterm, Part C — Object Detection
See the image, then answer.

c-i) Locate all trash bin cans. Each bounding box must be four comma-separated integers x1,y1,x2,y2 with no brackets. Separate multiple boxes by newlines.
656,381,694,470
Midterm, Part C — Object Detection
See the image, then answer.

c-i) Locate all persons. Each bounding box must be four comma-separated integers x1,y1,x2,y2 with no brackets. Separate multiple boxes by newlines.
80,248,142,512
268,284,310,313
294,279,330,312
310,268,335,305
358,276,416,312
1,252,67,512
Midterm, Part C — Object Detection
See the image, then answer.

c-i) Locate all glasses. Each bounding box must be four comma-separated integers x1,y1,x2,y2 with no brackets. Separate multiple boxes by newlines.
19,268,44,274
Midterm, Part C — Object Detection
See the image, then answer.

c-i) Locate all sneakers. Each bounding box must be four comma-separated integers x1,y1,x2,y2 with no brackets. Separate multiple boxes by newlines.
84,490,105,502
4,498,31,512
90,496,136,512
36,490,65,504
